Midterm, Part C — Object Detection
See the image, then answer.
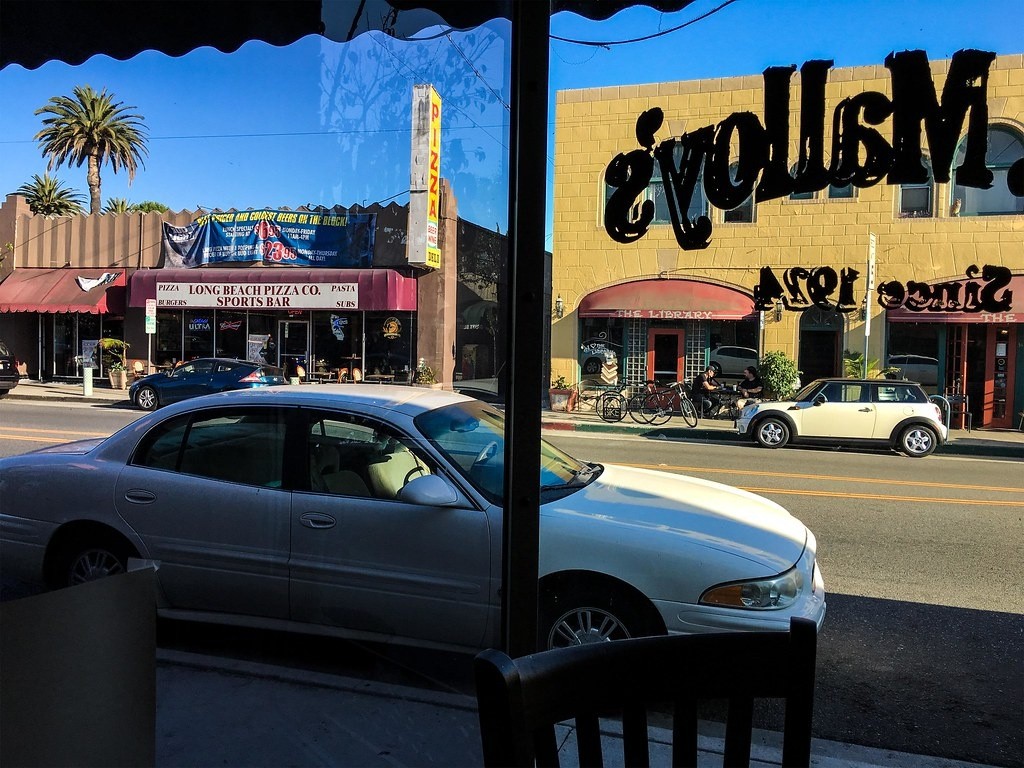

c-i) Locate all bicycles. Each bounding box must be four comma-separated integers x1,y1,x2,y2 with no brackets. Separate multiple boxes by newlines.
640,375,699,427
595,376,659,424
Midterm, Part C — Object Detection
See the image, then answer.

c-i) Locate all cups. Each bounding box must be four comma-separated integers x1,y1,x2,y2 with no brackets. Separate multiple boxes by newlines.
721,384,724,388
733,385,737,391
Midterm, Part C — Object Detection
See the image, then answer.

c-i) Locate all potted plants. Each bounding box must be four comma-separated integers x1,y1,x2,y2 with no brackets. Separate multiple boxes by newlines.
412,364,442,389
548,374,577,411
108,361,127,389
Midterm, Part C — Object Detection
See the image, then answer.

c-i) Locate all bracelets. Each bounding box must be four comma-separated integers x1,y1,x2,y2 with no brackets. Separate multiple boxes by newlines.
747,389,749,392
716,386,718,390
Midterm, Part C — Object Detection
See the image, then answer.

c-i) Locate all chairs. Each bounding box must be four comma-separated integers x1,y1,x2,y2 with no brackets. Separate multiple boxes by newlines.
296,366,311,384
310,444,371,497
684,381,710,419
326,368,348,384
132,359,148,380
472,616,818,768
367,433,431,499
942,393,972,434
353,368,362,384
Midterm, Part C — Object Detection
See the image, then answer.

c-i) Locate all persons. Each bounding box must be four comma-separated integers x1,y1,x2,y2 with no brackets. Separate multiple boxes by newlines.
735,365,765,421
688,365,721,419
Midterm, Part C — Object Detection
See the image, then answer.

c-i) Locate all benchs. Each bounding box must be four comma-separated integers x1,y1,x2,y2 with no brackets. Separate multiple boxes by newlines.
161,432,284,485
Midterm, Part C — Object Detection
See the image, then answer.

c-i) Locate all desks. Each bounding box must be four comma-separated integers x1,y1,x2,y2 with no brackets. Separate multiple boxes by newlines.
307,372,335,384
145,365,174,373
366,375,395,384
711,391,741,421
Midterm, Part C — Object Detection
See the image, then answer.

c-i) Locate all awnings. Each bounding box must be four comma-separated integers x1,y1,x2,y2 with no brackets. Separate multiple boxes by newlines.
573,280,758,326
1,266,134,316
887,272,1024,324
125,261,421,313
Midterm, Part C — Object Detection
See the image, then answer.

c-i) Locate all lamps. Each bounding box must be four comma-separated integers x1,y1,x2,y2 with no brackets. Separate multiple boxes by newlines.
777,297,783,321
554,292,563,318
860,298,866,320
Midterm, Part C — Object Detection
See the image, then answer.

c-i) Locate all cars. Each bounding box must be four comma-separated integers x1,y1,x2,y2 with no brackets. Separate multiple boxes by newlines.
0,341,20,394
128,357,284,412
887,355,940,385
0,379,827,718
710,345,759,377
737,377,948,458
579,345,605,373
